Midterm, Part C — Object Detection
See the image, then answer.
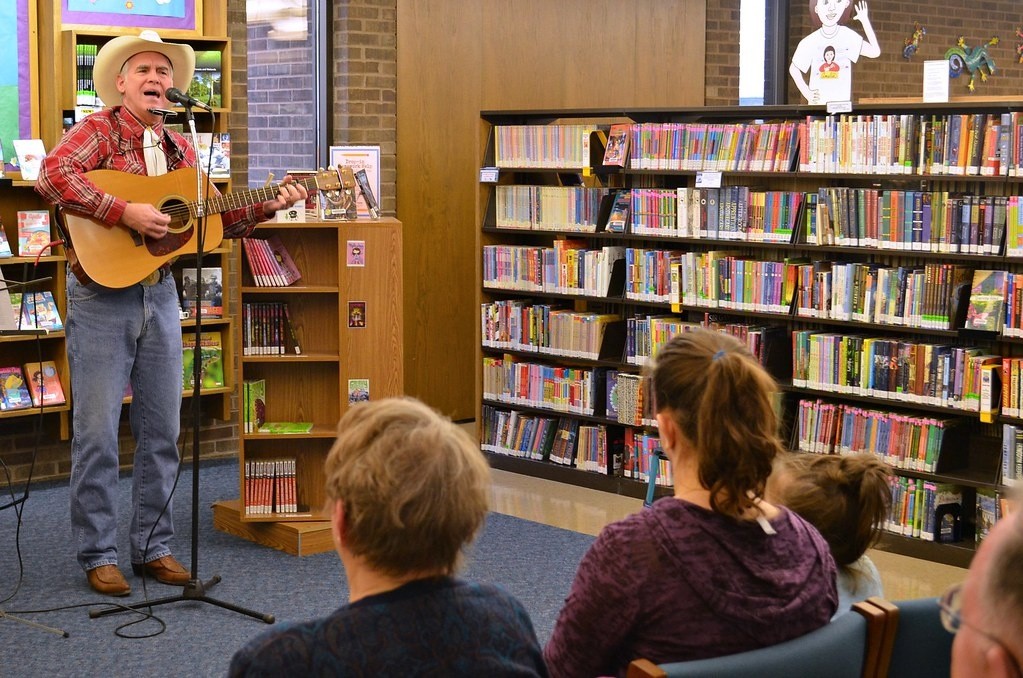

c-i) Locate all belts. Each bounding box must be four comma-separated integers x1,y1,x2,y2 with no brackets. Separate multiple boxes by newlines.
141,268,170,286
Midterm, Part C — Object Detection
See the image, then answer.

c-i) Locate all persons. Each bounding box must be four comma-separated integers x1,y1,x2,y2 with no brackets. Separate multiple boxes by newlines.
36,31,308,594
765,455,893,623
951,483,1023,678
545,330,839,678
228,398,547,678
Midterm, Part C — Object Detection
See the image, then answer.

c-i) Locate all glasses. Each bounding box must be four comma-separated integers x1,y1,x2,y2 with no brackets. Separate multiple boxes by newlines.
936,583,1023,678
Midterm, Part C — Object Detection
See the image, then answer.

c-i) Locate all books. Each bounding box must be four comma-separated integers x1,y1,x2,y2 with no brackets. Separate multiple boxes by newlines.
161,124,231,179
62,117,73,138
241,237,301,286
242,303,301,355
175,50,222,107
245,457,297,513
0,211,51,258
125,330,224,394
243,380,313,433
276,169,384,223
76,44,105,106
0,360,66,411
482,110,1022,547
183,269,223,319
0,139,47,180
10,291,63,332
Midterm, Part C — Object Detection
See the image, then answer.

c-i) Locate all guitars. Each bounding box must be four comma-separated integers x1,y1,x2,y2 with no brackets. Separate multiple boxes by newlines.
52,165,357,297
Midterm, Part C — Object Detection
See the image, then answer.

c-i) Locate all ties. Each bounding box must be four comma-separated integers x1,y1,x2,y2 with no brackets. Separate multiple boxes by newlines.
143,127,168,176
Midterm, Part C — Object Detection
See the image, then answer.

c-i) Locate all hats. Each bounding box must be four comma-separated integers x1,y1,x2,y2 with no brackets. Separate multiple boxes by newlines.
92,30,196,108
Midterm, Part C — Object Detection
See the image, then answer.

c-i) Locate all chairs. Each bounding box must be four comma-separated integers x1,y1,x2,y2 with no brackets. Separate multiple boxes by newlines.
624,601,885,678
870,589,966,678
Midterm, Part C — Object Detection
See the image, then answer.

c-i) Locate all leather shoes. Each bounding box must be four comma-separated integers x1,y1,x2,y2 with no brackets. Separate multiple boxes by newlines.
87,564,131,596
132,555,192,586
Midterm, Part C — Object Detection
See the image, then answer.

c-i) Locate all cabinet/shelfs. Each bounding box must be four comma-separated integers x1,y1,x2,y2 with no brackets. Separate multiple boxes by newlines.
1,32,242,442
474,93,1023,577
213,215,404,557
